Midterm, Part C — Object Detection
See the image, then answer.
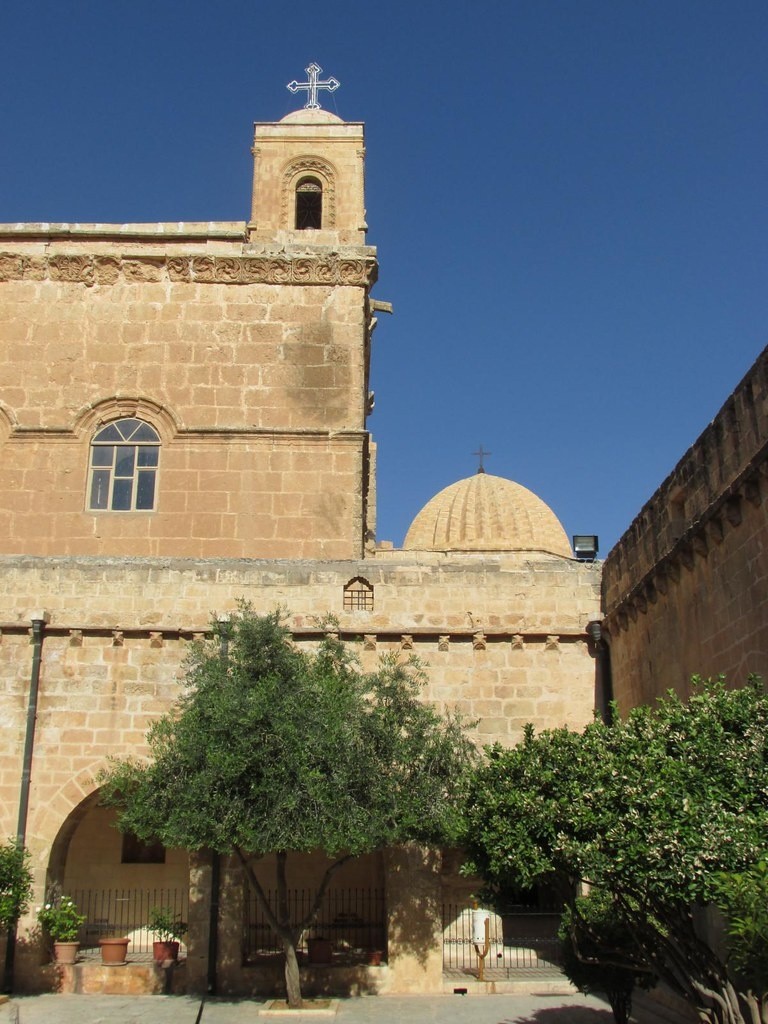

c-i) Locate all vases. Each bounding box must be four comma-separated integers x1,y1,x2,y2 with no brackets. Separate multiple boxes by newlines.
365,948,385,967
99,936,131,968
54,942,80,965
306,936,337,963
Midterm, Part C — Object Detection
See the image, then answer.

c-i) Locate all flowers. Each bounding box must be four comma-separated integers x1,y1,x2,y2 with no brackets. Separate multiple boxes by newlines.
35,895,88,943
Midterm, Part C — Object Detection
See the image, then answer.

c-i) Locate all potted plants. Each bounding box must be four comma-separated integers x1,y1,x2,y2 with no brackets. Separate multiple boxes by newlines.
137,905,189,963
